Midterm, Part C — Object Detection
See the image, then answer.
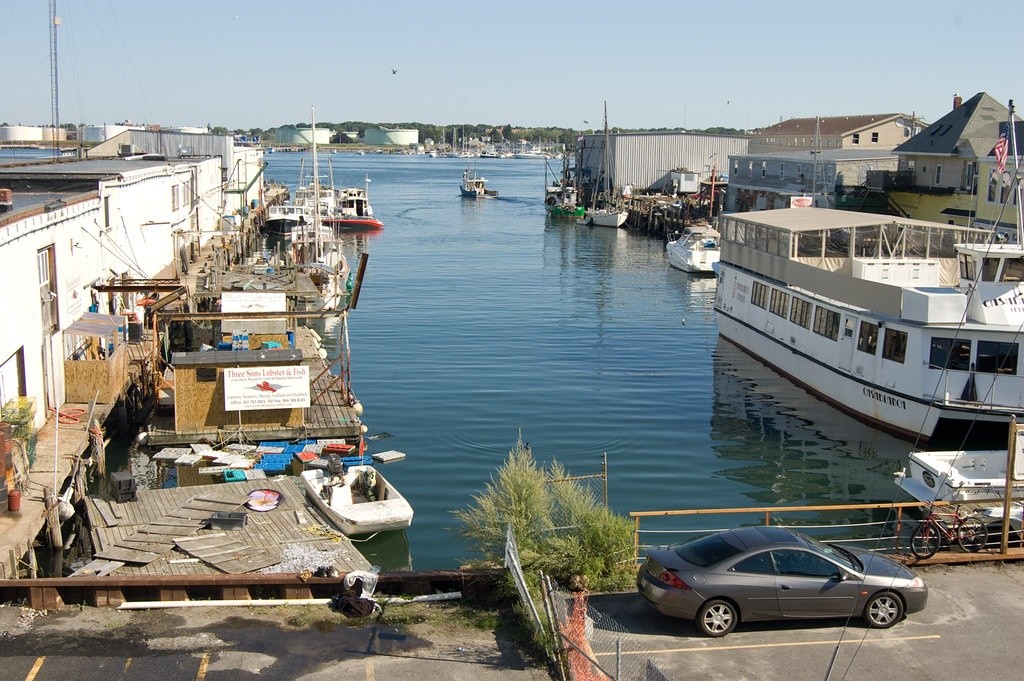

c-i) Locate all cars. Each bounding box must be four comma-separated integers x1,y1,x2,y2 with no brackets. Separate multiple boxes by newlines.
636,526,927,638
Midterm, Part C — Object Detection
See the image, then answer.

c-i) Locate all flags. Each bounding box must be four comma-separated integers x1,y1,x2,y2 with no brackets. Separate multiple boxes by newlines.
994,133,1009,171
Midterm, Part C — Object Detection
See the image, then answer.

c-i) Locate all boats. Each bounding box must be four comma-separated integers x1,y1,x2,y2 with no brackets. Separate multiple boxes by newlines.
357,149,363,156
331,149,336,153
895,450,1024,501
263,104,385,313
300,453,414,536
711,99,1024,447
460,161,499,199
973,500,1024,542
667,224,721,273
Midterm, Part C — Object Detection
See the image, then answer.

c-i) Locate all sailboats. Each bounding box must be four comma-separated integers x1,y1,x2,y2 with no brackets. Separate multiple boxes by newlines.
550,100,628,229
429,126,562,159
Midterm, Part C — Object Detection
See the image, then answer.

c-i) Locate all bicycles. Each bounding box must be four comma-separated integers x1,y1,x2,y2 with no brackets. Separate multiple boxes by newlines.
909,499,988,560
